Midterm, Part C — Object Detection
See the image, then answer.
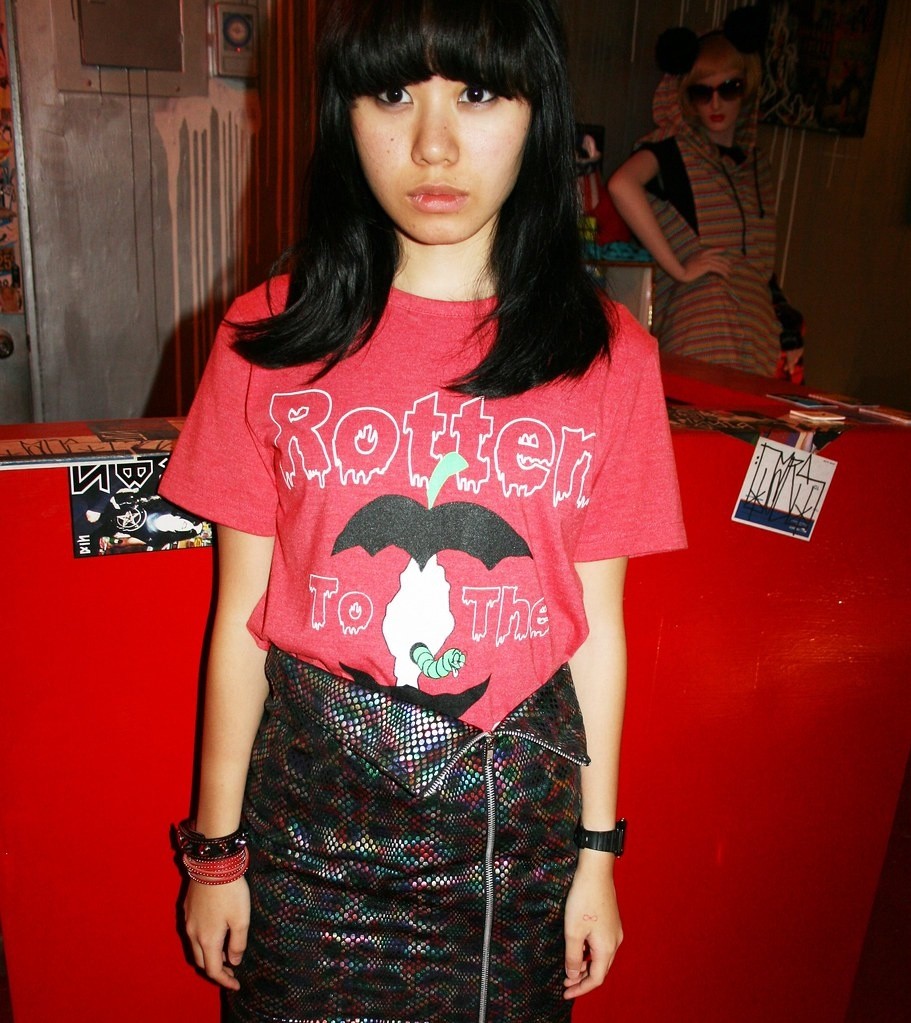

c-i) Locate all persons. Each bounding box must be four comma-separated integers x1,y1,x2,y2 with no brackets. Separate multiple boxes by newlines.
159,0,689,1023
608,5,806,384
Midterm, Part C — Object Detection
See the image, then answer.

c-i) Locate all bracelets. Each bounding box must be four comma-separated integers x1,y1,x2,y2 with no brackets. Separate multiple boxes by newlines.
175,819,256,885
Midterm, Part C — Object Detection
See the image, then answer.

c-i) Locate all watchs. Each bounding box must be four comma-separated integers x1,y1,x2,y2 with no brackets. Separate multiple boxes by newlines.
573,816,628,860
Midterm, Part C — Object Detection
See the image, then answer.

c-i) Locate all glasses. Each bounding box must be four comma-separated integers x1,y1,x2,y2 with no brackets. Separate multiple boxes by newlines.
687,68,747,105
